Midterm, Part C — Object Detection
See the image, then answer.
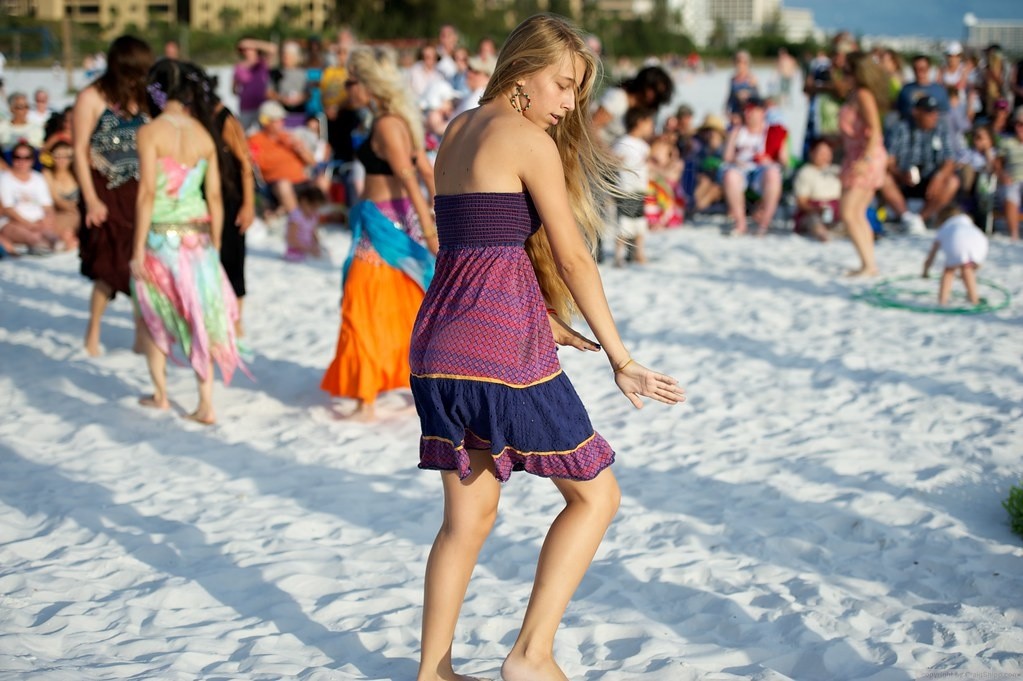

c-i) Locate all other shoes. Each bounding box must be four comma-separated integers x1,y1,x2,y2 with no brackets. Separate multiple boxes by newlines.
902,212,927,237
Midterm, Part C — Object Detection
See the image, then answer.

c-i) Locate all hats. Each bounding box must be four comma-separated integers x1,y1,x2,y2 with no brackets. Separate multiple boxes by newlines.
45,130,73,152
258,99,286,127
916,96,939,113
943,40,963,58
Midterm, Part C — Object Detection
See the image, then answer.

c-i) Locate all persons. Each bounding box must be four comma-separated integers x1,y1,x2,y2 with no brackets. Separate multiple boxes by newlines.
923,204,988,305
0,24,1023,256
408,13,687,681
71,34,145,358
130,58,257,423
830,67,886,275
181,59,256,338
611,106,651,266
320,44,439,423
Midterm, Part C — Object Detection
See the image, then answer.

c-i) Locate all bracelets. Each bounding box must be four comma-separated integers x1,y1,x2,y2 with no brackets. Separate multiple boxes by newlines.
547,308,557,315
614,359,633,373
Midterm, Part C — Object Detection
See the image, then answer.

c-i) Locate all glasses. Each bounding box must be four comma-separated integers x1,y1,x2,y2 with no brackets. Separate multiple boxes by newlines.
651,85,664,100
13,104,30,111
14,153,33,161
